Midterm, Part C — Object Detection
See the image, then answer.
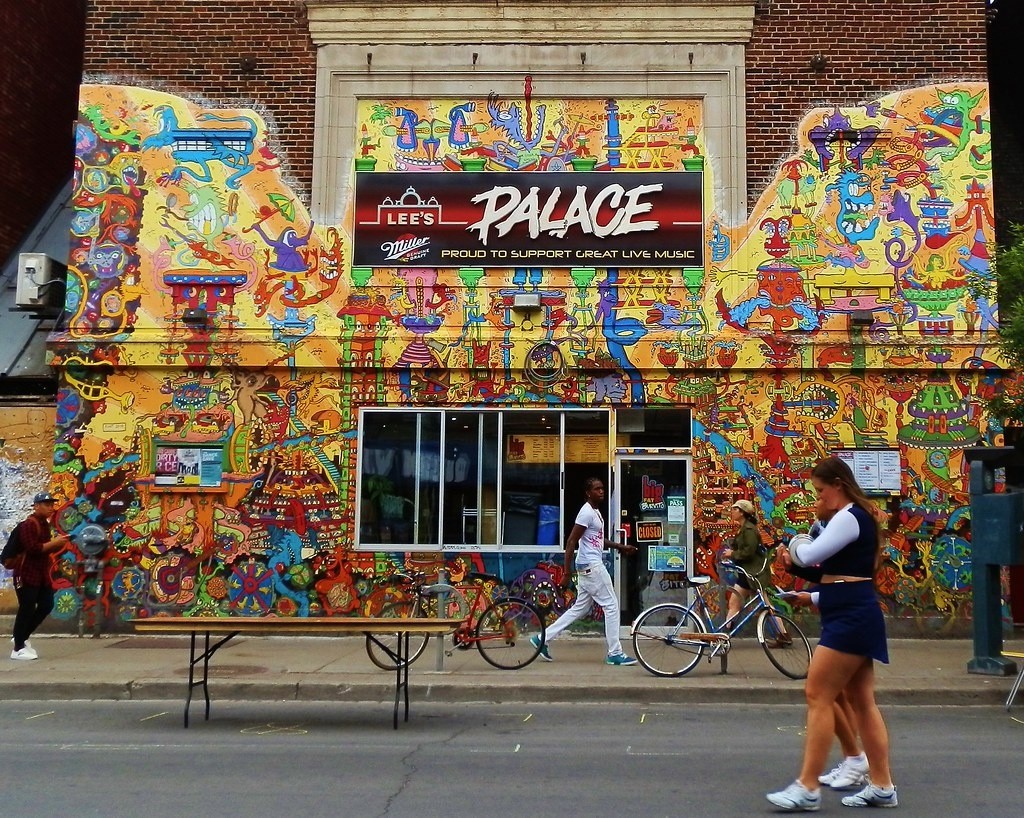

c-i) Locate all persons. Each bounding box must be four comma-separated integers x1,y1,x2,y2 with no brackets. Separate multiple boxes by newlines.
10,492,67,660
530,477,638,665
766,456,898,810
722,499,793,648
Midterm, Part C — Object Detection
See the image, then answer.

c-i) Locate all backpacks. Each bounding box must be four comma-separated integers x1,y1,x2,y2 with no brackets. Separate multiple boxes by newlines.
0,518,41,569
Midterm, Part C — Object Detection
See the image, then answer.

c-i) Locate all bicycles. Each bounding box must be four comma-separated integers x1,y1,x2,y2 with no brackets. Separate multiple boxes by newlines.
365,568,547,671
630,544,813,679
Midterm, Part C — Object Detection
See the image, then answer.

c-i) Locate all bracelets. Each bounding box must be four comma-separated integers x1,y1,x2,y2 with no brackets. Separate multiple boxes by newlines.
562,573,570,576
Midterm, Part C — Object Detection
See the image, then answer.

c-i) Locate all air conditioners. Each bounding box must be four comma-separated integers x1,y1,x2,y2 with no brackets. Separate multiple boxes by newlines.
16,254,67,306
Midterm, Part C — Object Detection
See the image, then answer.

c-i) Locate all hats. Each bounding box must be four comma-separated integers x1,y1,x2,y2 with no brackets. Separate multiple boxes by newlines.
34,492,59,502
731,499,756,514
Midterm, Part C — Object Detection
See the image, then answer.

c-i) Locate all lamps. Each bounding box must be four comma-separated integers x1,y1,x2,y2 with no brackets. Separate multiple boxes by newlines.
512,292,541,311
852,311,874,326
182,308,207,324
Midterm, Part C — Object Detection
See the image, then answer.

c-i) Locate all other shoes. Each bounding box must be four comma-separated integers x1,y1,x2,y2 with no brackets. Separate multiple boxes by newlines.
765,639,792,648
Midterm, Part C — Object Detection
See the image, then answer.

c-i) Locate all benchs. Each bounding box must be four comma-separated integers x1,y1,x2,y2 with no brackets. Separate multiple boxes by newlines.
128,616,467,729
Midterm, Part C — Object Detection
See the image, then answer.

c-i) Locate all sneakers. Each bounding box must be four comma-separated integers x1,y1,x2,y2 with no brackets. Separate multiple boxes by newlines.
606,653,637,666
766,779,821,810
818,763,864,786
10,648,38,659
842,774,898,807
529,635,553,661
831,752,870,788
11,637,32,647
